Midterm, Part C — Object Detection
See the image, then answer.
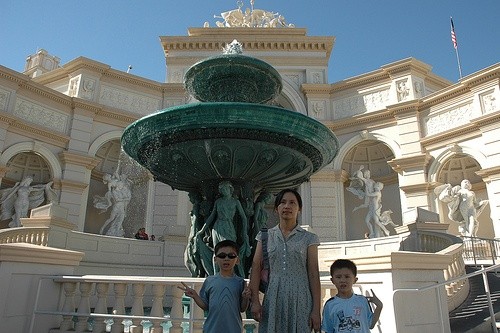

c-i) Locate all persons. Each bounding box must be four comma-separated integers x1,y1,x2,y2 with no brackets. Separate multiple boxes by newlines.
352,170,390,238
177,240,254,333
184,190,202,278
254,191,274,234
198,181,247,277
151,235,155,241
135,227,148,240
322,258,383,333
248,188,321,333
100,174,131,236
214,0,285,28
454,179,486,238
0,175,53,227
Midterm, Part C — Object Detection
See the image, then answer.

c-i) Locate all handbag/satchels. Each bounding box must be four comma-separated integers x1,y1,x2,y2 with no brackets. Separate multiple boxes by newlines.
256,228,271,293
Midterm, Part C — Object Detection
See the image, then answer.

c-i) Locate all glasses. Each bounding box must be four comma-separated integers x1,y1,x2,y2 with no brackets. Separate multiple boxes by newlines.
215,253,237,259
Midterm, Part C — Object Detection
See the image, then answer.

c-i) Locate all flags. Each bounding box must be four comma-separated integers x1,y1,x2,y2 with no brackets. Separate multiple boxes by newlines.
450,19,457,47
